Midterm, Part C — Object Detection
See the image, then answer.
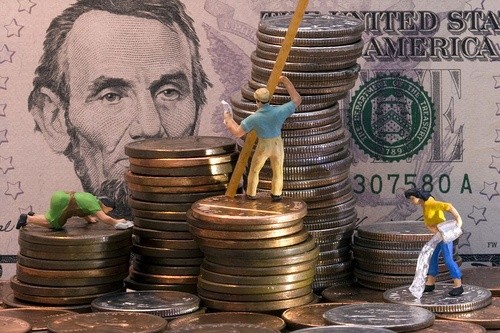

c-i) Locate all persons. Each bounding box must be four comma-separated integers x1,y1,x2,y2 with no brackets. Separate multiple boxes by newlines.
404,189,465,295
15,191,126,231
223,76,301,201
